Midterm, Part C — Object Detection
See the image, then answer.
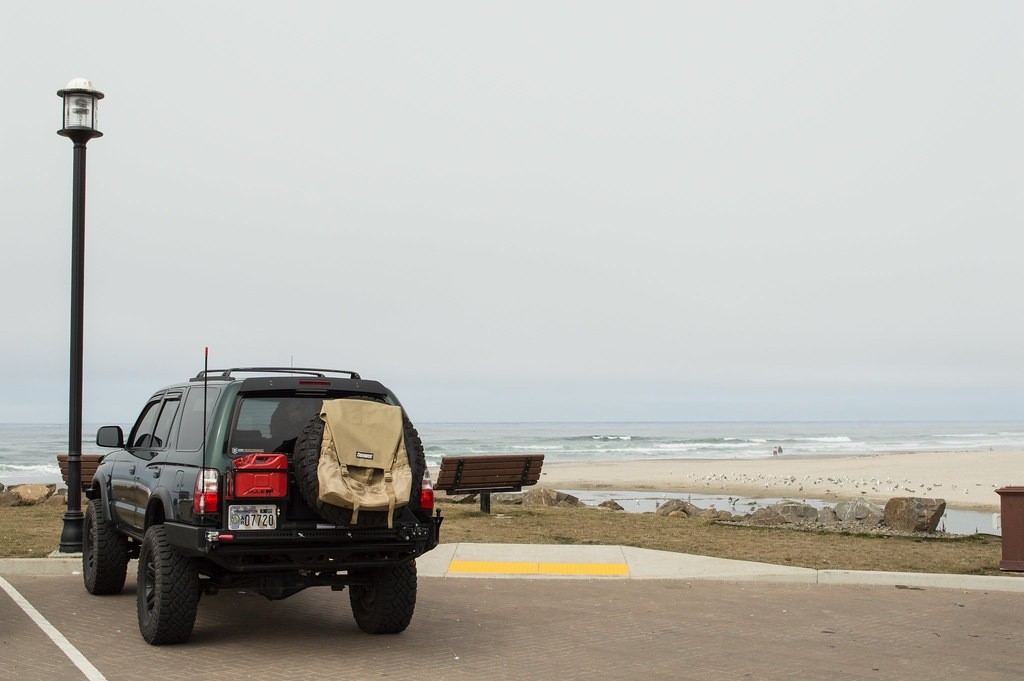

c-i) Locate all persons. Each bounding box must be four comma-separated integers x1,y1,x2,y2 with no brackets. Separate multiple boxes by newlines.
778,446,782,453
773,447,777,456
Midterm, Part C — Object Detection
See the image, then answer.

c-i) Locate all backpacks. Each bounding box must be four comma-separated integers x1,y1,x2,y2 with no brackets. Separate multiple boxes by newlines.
318,399,413,528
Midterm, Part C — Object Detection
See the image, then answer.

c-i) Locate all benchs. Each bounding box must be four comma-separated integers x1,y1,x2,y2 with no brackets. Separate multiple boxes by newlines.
433,452,544,514
57,453,106,493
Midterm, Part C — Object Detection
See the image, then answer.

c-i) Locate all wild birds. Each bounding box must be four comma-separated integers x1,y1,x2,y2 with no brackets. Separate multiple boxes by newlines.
728,497,740,506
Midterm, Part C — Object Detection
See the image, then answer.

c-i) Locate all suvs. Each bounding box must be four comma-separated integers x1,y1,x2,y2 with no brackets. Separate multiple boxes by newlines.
79,366,445,646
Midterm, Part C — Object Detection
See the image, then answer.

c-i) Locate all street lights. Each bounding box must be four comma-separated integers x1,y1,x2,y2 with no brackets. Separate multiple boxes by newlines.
54,77,107,554
